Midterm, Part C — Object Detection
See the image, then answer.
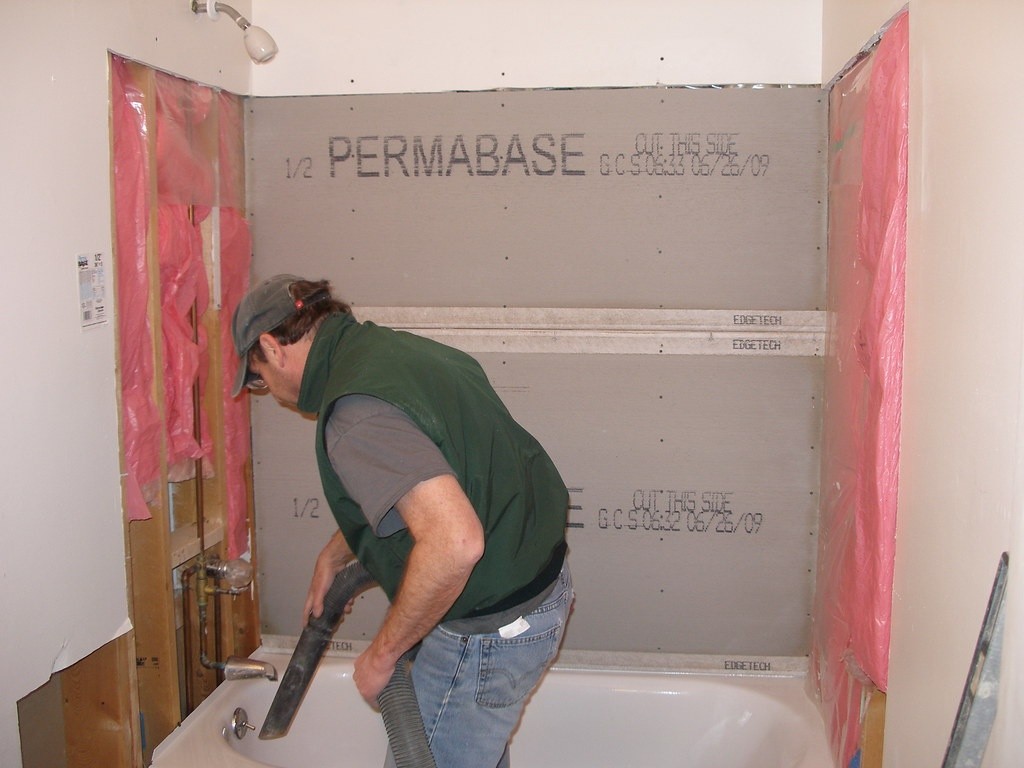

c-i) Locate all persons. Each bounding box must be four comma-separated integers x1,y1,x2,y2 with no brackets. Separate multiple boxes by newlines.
232,272,572,767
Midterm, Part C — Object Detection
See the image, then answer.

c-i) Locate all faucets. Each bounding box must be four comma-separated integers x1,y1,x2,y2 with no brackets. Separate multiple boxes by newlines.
222,655,279,682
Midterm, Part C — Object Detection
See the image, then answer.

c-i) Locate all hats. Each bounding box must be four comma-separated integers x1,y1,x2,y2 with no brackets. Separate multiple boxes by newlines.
230,273,331,399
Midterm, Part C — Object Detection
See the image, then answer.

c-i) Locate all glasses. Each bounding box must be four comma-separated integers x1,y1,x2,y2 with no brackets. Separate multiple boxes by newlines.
246,351,268,390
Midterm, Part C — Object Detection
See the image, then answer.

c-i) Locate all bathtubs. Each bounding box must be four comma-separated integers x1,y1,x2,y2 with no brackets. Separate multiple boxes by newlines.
147,638,840,768
